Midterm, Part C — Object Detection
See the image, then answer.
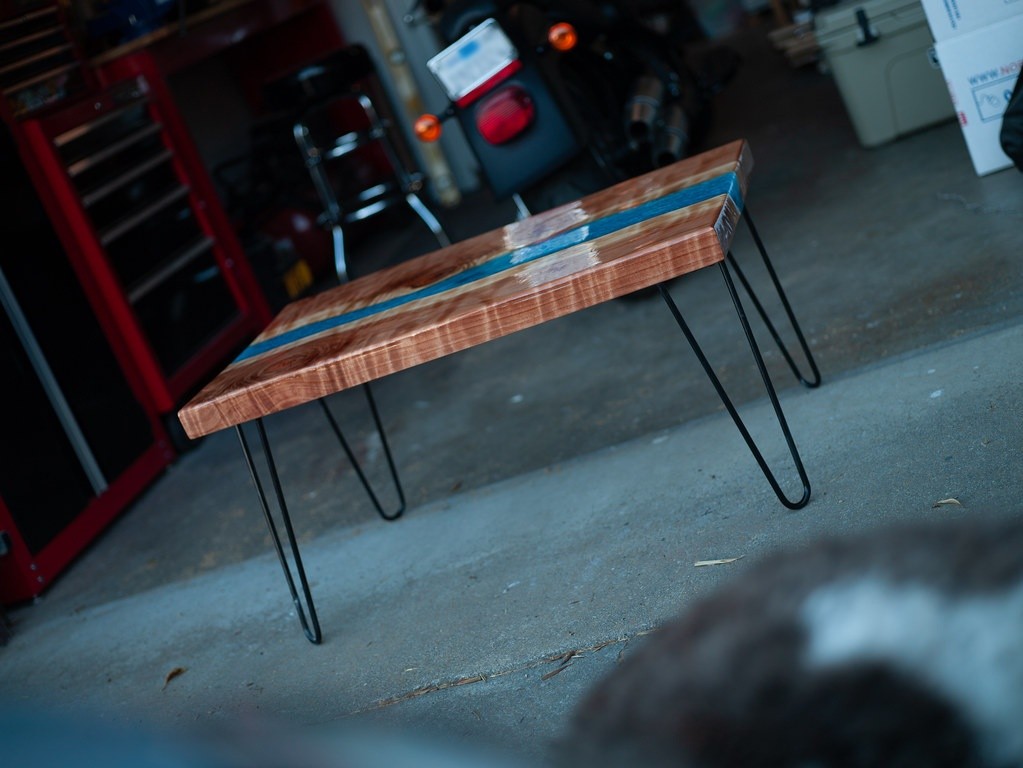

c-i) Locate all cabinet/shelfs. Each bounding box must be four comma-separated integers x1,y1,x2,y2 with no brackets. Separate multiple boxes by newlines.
0,0,404,612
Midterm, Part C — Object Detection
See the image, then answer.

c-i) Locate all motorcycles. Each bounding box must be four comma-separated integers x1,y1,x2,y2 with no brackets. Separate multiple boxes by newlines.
405,0,715,304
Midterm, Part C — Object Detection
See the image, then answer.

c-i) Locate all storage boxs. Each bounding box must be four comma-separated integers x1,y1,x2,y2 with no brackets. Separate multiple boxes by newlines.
813,0,1023,177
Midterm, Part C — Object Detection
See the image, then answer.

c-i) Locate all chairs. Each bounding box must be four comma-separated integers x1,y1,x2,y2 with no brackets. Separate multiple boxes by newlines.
254,40,452,284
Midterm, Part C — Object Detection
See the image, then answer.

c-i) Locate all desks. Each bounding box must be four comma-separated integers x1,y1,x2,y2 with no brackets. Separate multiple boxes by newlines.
177,138,822,645
